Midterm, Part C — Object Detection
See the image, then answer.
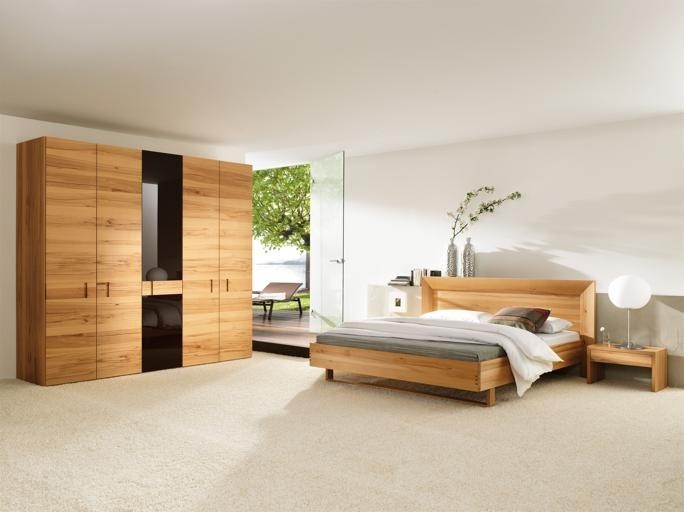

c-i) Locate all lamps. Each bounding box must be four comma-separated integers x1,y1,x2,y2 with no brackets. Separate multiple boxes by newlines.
606,273,653,350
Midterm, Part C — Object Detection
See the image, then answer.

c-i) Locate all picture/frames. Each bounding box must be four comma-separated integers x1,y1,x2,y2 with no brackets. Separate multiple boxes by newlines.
389,291,409,314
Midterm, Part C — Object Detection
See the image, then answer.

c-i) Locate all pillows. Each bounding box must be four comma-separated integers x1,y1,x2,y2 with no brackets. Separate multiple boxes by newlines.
487,307,551,333
538,316,574,335
420,308,487,322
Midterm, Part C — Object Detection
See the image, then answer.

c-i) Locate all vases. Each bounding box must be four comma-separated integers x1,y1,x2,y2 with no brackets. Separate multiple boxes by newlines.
447,238,477,277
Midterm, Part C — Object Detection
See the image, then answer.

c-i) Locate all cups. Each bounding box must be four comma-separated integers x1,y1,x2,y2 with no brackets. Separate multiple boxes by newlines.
602,331,610,348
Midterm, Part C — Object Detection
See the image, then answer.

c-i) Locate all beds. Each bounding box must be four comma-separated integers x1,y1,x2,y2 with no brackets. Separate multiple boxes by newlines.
309,277,596,406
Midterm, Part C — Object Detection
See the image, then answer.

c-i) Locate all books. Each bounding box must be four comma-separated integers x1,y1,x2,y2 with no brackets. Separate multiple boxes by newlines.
387,268,428,286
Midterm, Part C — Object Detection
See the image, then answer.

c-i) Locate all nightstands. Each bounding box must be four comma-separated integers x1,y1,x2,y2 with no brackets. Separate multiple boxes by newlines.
585,342,670,394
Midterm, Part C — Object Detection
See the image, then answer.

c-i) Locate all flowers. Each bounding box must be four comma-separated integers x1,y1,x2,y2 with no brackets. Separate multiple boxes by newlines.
447,184,521,238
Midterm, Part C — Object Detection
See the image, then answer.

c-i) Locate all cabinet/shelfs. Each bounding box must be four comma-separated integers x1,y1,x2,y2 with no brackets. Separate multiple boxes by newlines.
141,149,184,375
182,155,253,369
16,134,143,389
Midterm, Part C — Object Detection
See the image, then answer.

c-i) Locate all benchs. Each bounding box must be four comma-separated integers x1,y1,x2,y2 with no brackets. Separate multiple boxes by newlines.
252,282,304,320
